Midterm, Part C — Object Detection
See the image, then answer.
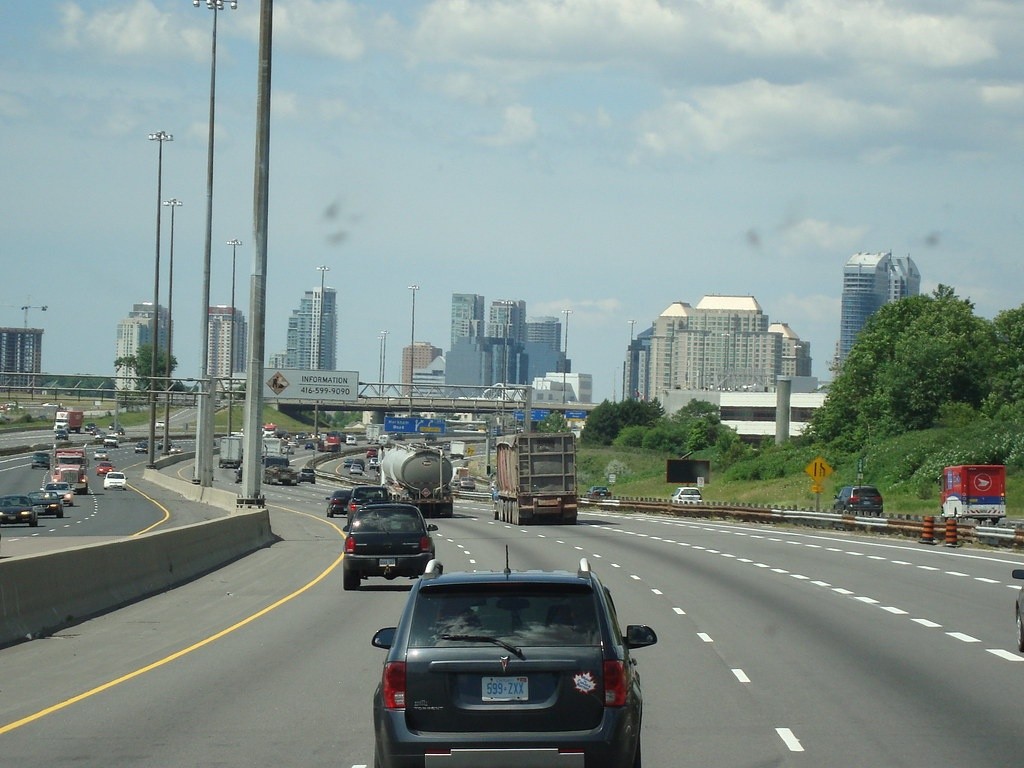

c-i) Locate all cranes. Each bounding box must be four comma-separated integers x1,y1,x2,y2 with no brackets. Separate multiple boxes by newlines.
21,294,49,328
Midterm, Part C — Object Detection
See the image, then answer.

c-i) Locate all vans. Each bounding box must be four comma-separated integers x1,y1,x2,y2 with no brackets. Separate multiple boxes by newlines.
378,435,391,445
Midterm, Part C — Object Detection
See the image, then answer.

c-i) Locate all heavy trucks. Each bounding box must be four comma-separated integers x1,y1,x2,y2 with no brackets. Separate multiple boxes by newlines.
51,443,90,496
487,432,579,526
262,444,298,487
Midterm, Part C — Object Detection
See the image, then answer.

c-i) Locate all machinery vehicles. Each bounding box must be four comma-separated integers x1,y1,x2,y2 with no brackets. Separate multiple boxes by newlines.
377,443,454,518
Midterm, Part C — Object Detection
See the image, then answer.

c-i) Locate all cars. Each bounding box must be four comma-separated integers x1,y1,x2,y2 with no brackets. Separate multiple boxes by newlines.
424,433,438,442
155,421,164,429
587,486,611,499
28,491,64,518
0,494,39,527
343,500,439,591
394,432,406,441
95,461,115,476
671,487,702,505
93,448,109,461
325,490,352,518
233,421,380,485
461,476,476,490
103,471,128,491
55,429,69,441
84,422,126,448
40,481,75,506
134,438,184,455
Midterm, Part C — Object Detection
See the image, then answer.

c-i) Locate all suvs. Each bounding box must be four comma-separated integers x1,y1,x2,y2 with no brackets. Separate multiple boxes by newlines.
833,485,883,517
346,486,388,525
371,556,659,767
30,452,51,470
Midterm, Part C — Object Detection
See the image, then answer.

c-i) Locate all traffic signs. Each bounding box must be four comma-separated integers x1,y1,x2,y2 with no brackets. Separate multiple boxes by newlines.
383,415,446,434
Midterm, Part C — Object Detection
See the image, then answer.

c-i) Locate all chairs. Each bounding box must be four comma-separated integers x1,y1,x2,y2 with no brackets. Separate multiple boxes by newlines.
4,501,11,506
434,602,479,644
358,492,367,499
374,492,382,499
544,602,595,639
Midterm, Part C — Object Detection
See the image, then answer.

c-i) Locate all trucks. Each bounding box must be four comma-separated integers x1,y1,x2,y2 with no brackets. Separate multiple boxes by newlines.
218,437,242,469
449,441,465,460
53,411,83,433
940,465,1006,526
451,466,469,487
366,423,389,444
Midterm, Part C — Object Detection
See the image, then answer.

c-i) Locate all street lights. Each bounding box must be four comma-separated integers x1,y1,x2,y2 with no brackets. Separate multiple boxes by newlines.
161,197,185,456
627,320,637,399
377,330,390,401
312,265,331,438
561,309,573,405
143,129,175,467
500,300,514,433
226,238,243,435
721,333,731,389
407,284,419,418
